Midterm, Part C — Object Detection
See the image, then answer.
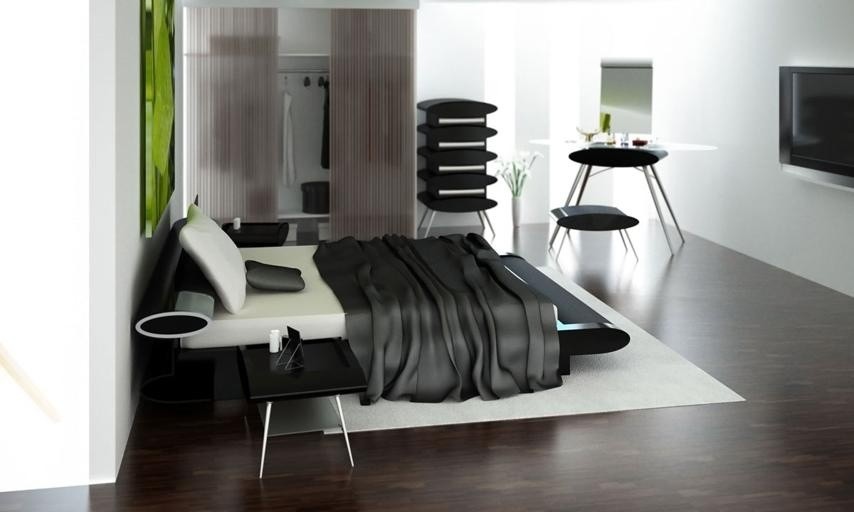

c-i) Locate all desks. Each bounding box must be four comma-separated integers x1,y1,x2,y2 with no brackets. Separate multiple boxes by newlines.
547,145,686,257
234,339,367,479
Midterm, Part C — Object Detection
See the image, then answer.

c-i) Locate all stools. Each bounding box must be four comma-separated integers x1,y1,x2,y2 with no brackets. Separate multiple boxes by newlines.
548,205,639,263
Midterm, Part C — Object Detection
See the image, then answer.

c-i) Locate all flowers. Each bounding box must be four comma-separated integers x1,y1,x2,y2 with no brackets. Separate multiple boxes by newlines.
493,151,543,197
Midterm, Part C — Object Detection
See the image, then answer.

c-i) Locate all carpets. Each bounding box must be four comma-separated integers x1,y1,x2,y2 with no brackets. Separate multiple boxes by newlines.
322,265,746,435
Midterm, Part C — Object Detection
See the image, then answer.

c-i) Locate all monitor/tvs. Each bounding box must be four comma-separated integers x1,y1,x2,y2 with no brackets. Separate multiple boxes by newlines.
779,66,854,178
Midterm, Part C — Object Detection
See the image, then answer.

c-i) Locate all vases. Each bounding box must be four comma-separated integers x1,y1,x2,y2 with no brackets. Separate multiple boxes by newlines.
511,197,523,228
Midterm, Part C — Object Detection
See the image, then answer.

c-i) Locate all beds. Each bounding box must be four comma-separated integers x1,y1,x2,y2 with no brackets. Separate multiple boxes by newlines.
132,216,629,405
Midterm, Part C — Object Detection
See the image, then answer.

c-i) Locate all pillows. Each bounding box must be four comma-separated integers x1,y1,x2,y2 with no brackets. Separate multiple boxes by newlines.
179,203,305,315
